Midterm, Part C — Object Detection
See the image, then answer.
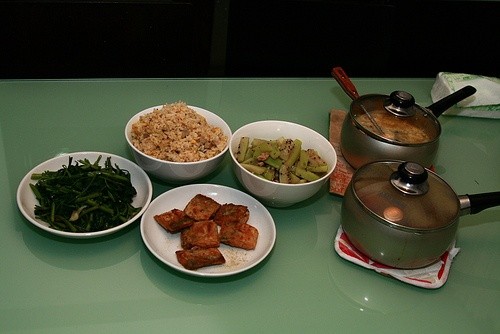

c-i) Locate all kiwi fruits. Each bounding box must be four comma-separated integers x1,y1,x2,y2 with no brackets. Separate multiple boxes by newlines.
236,136,329,185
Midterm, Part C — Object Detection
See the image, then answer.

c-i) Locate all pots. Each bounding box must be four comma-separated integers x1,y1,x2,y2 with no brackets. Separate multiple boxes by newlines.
340,85,477,171
341,160,500,270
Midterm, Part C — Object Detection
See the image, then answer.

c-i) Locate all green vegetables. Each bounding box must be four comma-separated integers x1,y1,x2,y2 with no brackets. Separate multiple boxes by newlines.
29,155,142,233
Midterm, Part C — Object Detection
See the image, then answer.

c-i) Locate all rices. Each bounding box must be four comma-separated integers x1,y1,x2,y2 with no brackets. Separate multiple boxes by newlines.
131,99,228,162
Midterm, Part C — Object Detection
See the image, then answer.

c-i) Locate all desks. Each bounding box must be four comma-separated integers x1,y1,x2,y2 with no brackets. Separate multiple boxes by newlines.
0,78,500,334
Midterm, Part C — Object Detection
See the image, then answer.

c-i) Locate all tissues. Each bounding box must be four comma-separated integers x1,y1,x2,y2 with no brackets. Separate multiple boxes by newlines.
429,72,500,116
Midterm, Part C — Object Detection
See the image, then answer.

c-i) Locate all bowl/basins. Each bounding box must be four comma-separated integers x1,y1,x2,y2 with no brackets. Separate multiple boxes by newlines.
229,120,337,207
124,104,232,181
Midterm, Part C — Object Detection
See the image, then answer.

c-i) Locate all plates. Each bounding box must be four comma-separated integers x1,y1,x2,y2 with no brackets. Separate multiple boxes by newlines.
140,184,276,277
16,152,153,238
